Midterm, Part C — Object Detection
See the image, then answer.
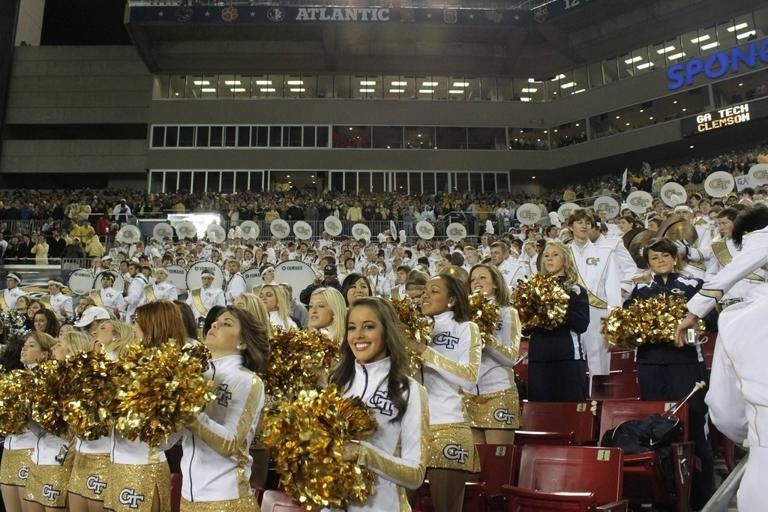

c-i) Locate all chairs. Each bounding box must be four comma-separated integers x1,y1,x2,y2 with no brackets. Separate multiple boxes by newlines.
167,473,319,511
597,399,701,512
507,332,738,473
424,443,517,512
501,444,629,512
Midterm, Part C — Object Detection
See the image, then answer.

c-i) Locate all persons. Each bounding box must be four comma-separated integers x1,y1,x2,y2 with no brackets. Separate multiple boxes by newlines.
104,299,183,512
465,263,522,446
67,318,136,512
326,296,429,512
0,145,768,403
0,332,57,511
704,297,768,511
24,332,91,511
624,235,716,512
231,294,273,492
159,308,267,512
407,272,482,512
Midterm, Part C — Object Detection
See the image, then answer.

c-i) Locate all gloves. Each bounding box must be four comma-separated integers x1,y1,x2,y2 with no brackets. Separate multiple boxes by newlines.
674,239,691,257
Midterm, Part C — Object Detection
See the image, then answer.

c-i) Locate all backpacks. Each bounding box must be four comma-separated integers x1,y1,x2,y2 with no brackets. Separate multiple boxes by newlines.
601,415,683,495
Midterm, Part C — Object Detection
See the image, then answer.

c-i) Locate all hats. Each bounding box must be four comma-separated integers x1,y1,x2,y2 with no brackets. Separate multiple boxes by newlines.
73,307,111,328
323,265,336,275
101,255,112,262
